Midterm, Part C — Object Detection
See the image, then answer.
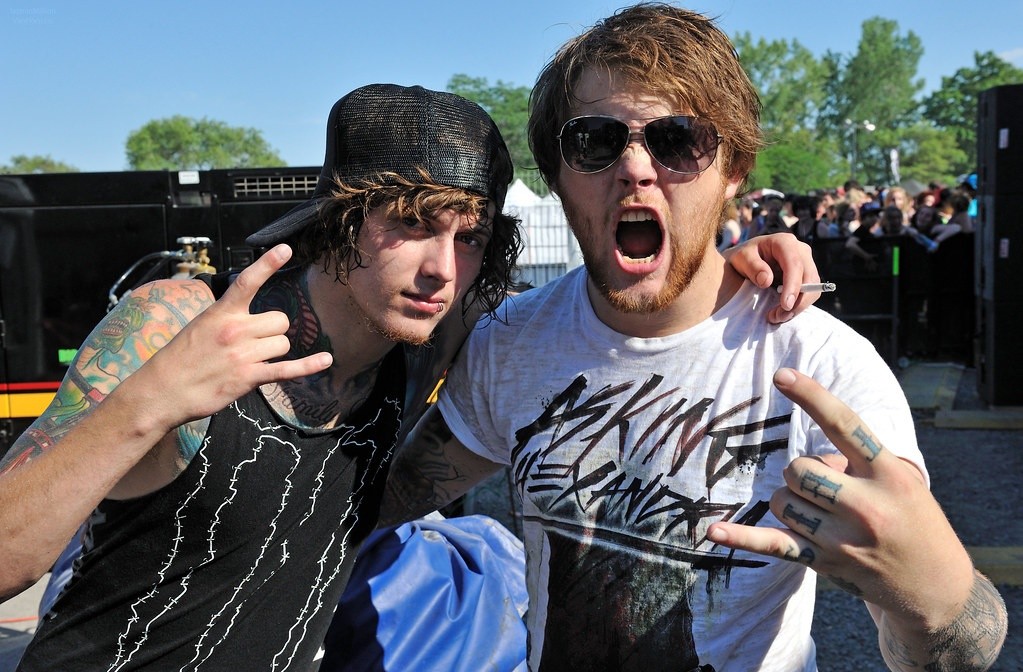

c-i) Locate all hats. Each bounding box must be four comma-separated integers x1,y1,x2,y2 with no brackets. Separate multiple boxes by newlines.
245,83,514,251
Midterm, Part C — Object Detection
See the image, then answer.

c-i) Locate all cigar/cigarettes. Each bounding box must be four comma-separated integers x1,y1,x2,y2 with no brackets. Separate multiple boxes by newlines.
777,283,836,293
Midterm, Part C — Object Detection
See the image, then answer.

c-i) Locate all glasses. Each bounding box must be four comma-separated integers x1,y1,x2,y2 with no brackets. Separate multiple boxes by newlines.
551,115,725,175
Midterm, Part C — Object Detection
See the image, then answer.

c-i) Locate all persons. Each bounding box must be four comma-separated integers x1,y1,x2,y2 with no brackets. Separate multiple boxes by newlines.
1,82,821,672
367,2,1006,672
718,174,978,362
